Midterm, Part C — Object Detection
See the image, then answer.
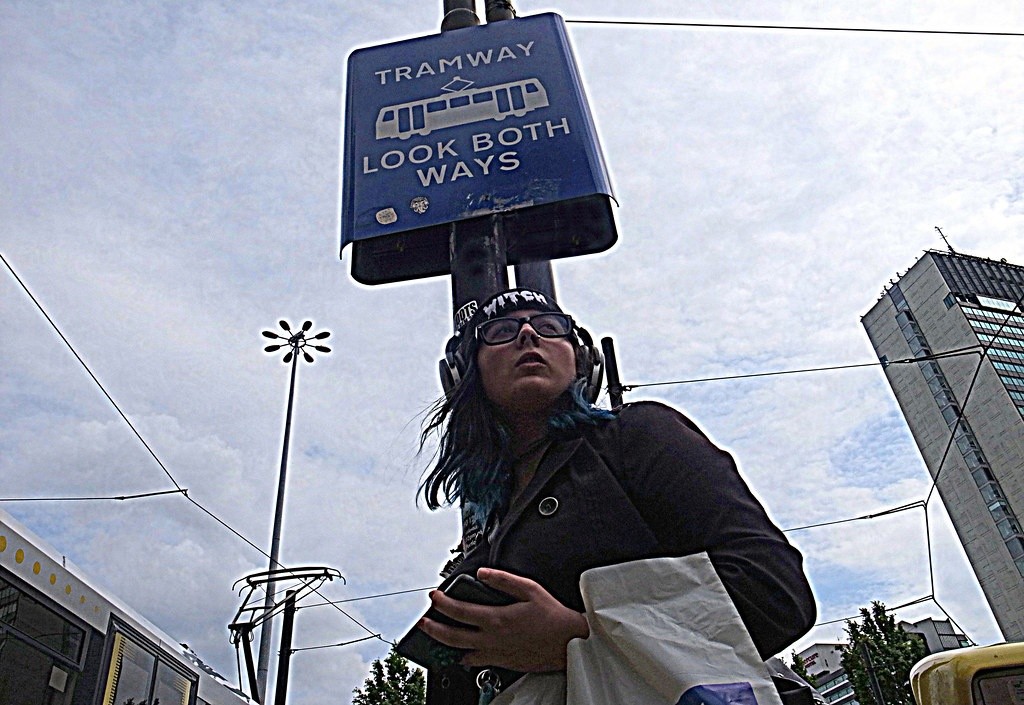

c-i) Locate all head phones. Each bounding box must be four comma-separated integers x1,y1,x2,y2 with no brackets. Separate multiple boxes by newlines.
439,319,605,405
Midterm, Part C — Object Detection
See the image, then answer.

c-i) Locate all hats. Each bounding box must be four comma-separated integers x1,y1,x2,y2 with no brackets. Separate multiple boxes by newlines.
458,288,566,340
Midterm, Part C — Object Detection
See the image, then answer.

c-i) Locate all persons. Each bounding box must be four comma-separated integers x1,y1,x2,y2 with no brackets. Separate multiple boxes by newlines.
391,286,817,705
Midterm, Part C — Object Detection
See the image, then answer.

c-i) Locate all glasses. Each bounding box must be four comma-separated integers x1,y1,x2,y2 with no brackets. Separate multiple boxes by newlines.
471,312,575,347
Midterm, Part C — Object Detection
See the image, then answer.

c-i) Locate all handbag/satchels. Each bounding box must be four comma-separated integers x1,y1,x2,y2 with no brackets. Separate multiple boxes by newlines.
485,552,783,705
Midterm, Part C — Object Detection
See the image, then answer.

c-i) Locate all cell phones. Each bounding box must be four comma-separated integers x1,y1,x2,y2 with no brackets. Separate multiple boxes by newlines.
396,574,511,672
